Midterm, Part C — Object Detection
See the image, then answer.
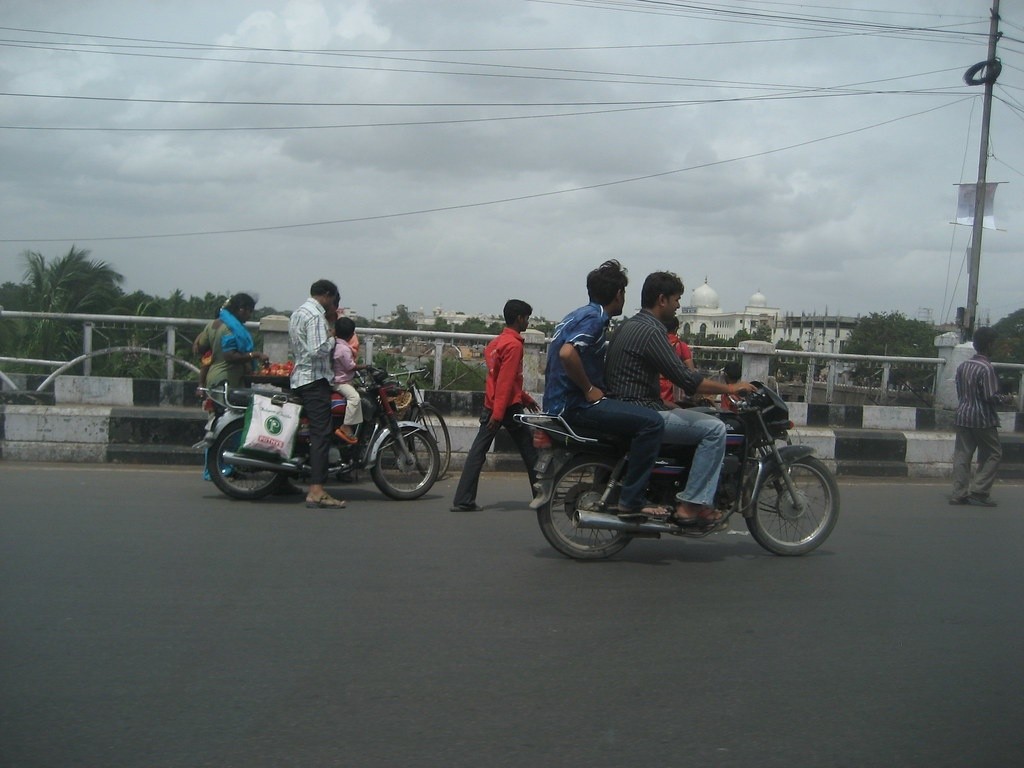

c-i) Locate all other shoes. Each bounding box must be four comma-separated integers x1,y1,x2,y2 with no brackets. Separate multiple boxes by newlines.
281,486,302,494
449,503,483,512
306,491,345,509
949,496,969,505
966,493,997,507
335,426,358,443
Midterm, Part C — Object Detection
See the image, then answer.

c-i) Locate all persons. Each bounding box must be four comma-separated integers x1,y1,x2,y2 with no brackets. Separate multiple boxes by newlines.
603,271,759,525
289,280,346,509
949,327,1014,507
192,293,269,481
721,361,741,411
543,260,674,518
659,316,694,401
449,299,541,512
332,317,366,443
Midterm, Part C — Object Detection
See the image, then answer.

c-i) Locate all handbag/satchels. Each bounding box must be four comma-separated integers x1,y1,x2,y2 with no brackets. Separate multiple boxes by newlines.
237,393,304,462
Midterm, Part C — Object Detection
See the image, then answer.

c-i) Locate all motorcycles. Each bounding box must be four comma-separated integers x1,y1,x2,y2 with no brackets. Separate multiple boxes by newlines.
195,365,441,501
512,379,840,561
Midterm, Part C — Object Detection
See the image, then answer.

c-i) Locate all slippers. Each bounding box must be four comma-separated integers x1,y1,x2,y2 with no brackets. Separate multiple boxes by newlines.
617,505,671,518
673,506,726,524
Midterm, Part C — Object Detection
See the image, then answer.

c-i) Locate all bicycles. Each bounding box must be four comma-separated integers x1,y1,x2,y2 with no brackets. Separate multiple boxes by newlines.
389,366,452,479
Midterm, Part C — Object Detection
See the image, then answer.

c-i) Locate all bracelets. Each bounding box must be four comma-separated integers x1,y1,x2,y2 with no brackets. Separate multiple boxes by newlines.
583,384,593,392
729,384,734,394
249,352,253,359
